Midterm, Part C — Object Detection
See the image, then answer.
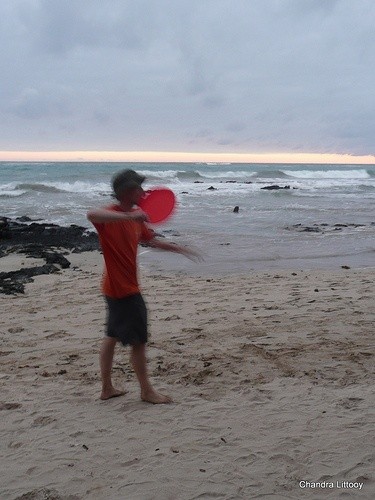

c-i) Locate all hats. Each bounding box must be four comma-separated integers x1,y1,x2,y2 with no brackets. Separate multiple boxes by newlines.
110,170,146,197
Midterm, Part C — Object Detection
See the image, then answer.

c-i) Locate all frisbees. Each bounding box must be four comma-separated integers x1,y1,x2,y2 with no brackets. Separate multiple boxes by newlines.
138,189,176,224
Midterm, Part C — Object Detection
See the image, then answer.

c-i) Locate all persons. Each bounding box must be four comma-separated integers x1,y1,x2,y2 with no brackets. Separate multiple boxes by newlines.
84,169,206,406
